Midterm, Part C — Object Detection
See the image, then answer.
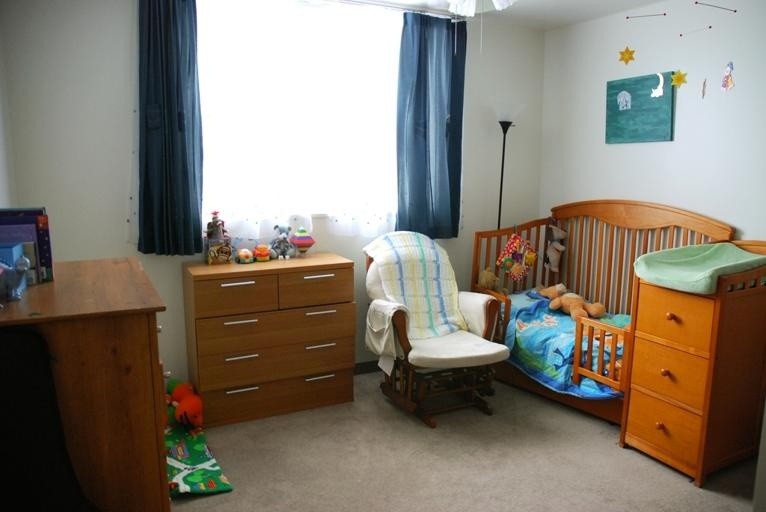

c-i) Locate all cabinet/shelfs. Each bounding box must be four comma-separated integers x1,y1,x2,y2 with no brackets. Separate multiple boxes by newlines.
618,237,766,487
2,255,171,510
180,253,359,433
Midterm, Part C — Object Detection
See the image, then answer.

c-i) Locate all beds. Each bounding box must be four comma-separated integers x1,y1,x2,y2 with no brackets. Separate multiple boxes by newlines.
470,197,737,431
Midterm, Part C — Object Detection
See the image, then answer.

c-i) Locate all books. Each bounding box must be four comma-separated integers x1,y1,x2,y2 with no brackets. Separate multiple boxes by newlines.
0,207,53,285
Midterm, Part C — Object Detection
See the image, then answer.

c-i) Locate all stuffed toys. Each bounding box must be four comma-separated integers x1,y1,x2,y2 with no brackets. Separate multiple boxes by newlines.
270,225,296,259
203,211,271,265
164,377,204,427
287,225,316,257
478,224,606,320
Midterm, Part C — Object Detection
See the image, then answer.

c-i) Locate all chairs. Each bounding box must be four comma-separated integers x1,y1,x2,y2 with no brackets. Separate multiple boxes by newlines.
363,241,503,429
